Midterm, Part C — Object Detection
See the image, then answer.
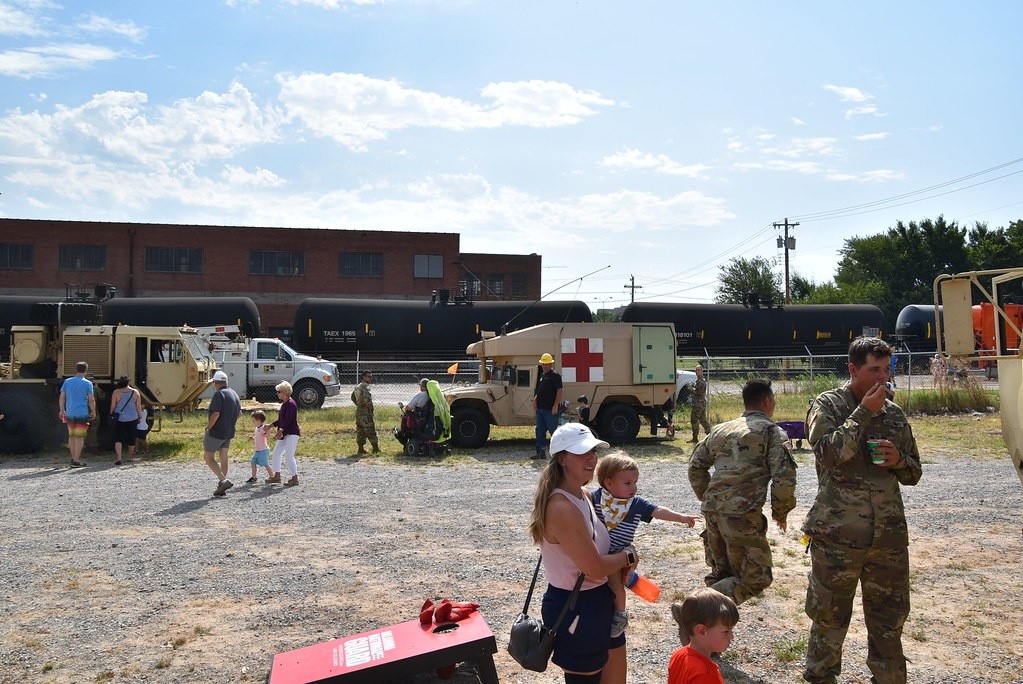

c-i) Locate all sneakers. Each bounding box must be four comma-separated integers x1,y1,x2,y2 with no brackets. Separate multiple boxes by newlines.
610,609,629,638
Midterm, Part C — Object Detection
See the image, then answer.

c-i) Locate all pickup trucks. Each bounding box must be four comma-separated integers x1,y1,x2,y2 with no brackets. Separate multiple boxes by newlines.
198,324,342,410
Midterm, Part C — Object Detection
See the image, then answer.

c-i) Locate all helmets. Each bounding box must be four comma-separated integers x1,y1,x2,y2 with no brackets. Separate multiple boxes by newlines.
539,353,554,364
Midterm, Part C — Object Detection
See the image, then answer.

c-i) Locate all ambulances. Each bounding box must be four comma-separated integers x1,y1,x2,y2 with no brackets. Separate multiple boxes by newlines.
442,319,678,450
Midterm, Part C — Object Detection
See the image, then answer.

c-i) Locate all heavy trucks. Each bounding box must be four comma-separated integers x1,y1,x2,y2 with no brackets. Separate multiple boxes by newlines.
0,322,222,454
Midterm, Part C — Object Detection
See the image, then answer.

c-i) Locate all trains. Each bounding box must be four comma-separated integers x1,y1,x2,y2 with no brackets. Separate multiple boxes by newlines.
0,287,1023,368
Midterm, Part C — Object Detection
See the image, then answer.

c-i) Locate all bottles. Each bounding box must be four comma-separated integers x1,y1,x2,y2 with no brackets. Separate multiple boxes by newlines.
623,568,660,602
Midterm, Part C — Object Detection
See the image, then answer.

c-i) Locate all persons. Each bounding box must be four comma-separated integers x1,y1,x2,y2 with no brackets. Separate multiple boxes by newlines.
592,451,699,638
59,362,96,468
560,407,575,423
246,410,274,483
576,395,590,426
351,370,383,455
671,379,798,659
406,378,431,416
932,354,970,388
801,338,922,684
203,370,242,496
264,381,301,486
133,403,149,454
686,365,711,443
887,351,898,387
668,588,740,684
531,354,563,460
110,374,142,465
531,423,640,684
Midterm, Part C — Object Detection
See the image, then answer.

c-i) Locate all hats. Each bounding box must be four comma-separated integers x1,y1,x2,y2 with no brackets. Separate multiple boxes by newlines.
550,423,610,457
212,371,228,382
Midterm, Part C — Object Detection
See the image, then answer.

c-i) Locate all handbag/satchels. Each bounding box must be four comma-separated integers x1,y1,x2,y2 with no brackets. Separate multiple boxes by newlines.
106,413,119,431
508,613,556,672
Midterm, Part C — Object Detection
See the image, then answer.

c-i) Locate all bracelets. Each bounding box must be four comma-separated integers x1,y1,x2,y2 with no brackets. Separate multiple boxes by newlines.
625,547,636,562
205,427,209,431
279,428,283,431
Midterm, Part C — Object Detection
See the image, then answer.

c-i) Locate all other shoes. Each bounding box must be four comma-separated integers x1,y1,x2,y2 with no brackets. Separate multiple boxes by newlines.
672,425,675,436
358,445,366,453
666,432,672,436
246,477,257,483
124,459,133,462
671,603,690,646
372,444,380,453
70,459,87,467
141,449,150,454
686,435,698,443
214,479,233,495
134,449,139,454
114,460,121,465
211,494,228,499
893,384,896,388
265,477,281,485
283,480,299,486
530,454,546,459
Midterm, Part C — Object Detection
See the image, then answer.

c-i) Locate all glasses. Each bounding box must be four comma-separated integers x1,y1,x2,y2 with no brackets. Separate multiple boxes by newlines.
366,376,374,378
418,383,421,386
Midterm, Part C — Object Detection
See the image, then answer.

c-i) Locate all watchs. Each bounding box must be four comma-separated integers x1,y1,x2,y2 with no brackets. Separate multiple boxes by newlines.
623,549,635,568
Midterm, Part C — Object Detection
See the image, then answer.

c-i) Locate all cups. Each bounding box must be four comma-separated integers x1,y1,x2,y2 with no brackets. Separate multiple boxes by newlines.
866,439,889,464
